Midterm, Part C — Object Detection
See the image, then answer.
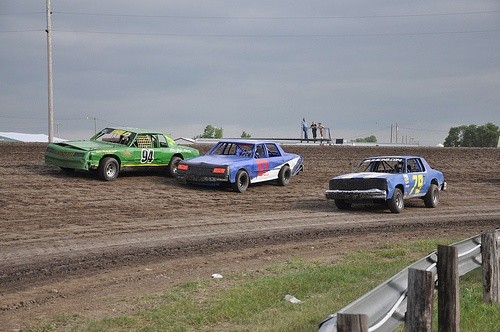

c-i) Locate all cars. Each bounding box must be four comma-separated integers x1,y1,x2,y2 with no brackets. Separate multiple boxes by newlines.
177,138,303,193
324,155,446,213
45,127,199,182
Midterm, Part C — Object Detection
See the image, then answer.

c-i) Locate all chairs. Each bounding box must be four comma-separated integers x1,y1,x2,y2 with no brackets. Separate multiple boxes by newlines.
394,163,411,172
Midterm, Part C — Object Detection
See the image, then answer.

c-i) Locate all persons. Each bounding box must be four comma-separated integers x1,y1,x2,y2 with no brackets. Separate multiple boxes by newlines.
300,118,325,139
